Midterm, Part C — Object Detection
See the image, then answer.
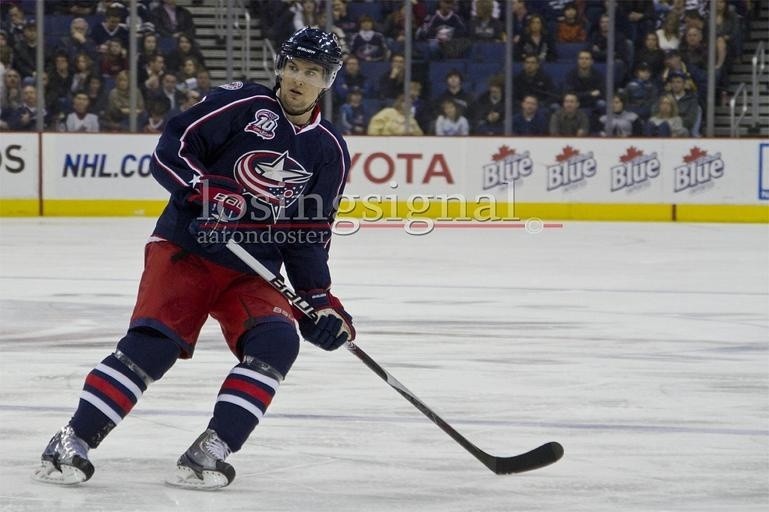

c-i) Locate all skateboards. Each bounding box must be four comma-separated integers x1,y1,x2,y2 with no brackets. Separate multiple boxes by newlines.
226,238,564,475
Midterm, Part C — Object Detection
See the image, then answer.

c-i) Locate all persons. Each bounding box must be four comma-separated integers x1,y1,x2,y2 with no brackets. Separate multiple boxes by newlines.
38,27,355,490
0,0,759,136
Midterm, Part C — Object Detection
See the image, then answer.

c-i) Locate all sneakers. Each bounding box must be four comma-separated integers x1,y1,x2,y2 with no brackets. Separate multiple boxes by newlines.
176,429,236,488
41,422,95,481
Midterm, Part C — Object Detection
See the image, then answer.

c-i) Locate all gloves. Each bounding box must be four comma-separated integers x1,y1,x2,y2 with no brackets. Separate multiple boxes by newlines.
291,288,357,352
187,174,243,253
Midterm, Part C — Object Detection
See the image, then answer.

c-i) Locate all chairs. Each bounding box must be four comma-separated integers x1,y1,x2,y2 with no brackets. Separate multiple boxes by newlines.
0,1,746,138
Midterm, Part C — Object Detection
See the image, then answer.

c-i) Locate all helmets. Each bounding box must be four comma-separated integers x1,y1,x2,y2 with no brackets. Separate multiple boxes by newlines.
274,25,345,92
